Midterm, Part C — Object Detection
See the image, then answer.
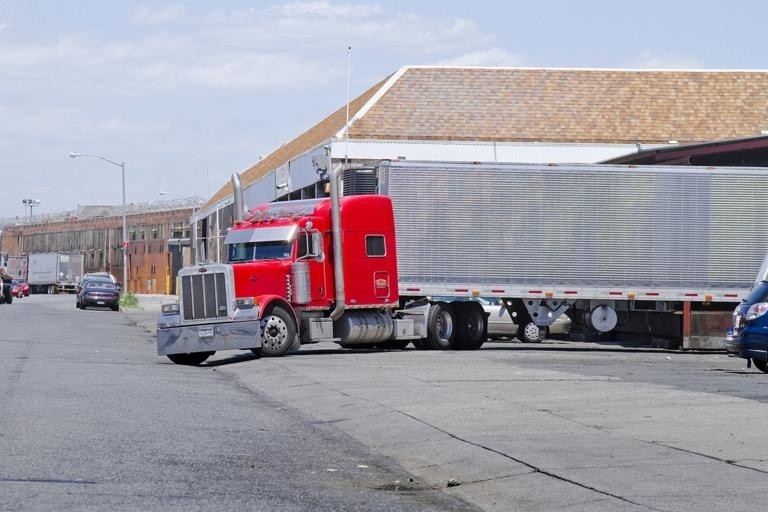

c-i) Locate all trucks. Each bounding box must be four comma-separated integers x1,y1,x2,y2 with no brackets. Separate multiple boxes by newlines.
18,251,85,294
154,154,768,367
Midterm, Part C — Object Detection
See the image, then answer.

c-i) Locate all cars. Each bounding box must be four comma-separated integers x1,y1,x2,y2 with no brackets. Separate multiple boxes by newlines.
76,270,122,311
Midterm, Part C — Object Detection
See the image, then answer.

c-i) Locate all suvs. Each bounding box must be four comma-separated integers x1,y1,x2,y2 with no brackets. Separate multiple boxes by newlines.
724,279,768,374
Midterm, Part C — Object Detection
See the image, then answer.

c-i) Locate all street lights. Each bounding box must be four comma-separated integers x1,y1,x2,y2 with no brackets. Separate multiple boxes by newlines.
69,151,129,300
159,190,195,213
